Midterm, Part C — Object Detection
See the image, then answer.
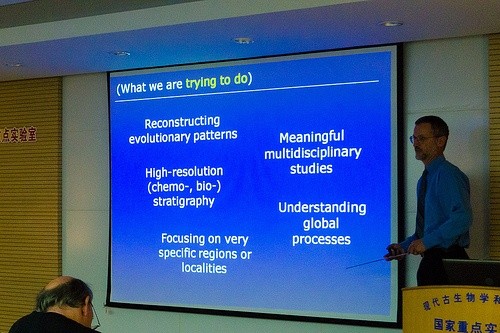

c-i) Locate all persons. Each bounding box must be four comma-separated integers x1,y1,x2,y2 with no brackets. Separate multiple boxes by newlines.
385,116,472,285
9,276,104,333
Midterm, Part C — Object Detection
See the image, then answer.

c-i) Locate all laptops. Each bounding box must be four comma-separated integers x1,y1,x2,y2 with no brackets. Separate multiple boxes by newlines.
442,259,500,287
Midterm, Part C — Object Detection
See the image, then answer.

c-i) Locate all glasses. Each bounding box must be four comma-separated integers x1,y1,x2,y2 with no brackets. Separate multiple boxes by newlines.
89,300,100,329
409,136,434,144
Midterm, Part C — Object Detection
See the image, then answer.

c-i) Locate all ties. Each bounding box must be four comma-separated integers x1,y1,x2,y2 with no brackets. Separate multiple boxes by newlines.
416,169,428,240
